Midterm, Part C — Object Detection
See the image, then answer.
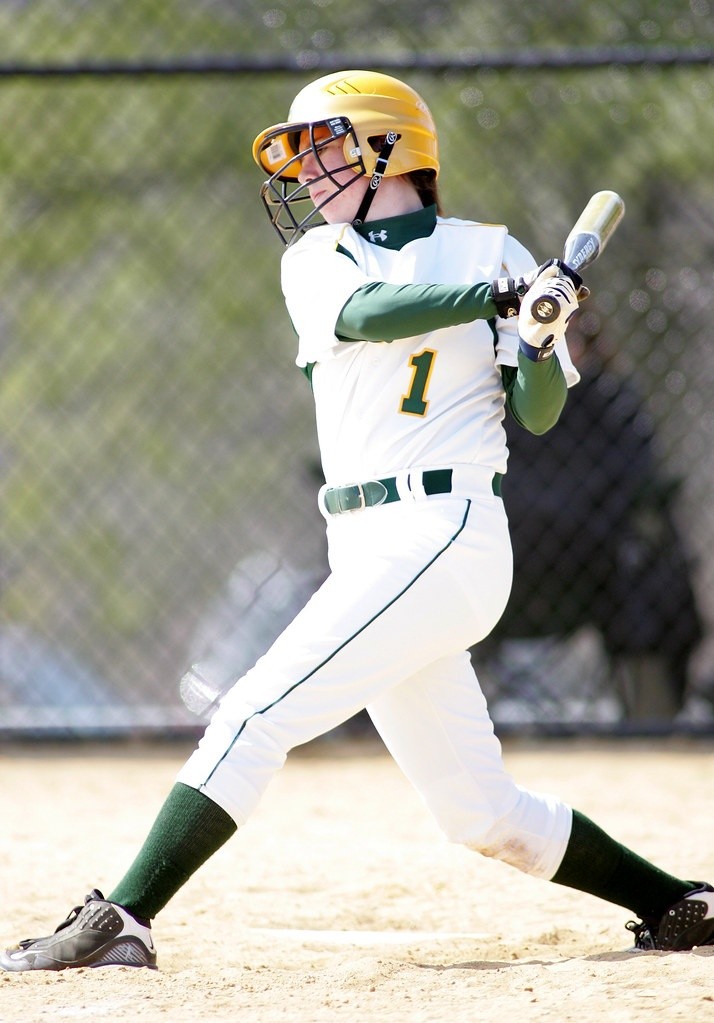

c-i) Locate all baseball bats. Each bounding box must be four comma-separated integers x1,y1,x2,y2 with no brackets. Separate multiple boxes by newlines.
530,190,624,325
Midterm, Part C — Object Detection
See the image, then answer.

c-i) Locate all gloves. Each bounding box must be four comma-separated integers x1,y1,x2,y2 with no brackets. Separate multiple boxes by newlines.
490,259,592,360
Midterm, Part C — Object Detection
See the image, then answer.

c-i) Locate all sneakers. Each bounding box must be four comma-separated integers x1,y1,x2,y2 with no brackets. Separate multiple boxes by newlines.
624,880,714,951
0,889,158,975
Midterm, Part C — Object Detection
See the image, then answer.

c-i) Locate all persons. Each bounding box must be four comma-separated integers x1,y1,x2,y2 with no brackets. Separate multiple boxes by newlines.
0,69,714,973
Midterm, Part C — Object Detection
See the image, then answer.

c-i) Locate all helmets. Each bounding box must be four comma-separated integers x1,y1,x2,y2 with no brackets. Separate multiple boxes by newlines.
252,70,440,247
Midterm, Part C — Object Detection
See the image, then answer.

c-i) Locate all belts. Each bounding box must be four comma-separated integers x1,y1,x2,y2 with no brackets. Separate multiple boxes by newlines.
320,470,504,513
